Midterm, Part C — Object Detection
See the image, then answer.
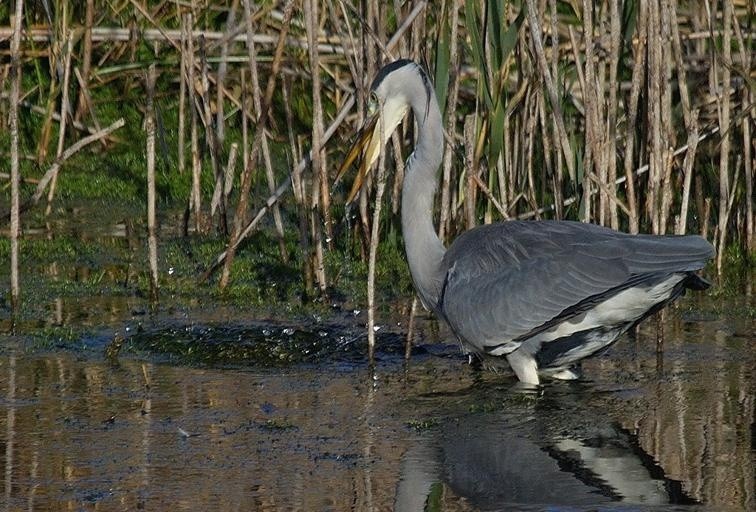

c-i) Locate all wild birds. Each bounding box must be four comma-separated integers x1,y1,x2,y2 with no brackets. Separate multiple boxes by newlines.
390,377,705,512
331,58,717,386
